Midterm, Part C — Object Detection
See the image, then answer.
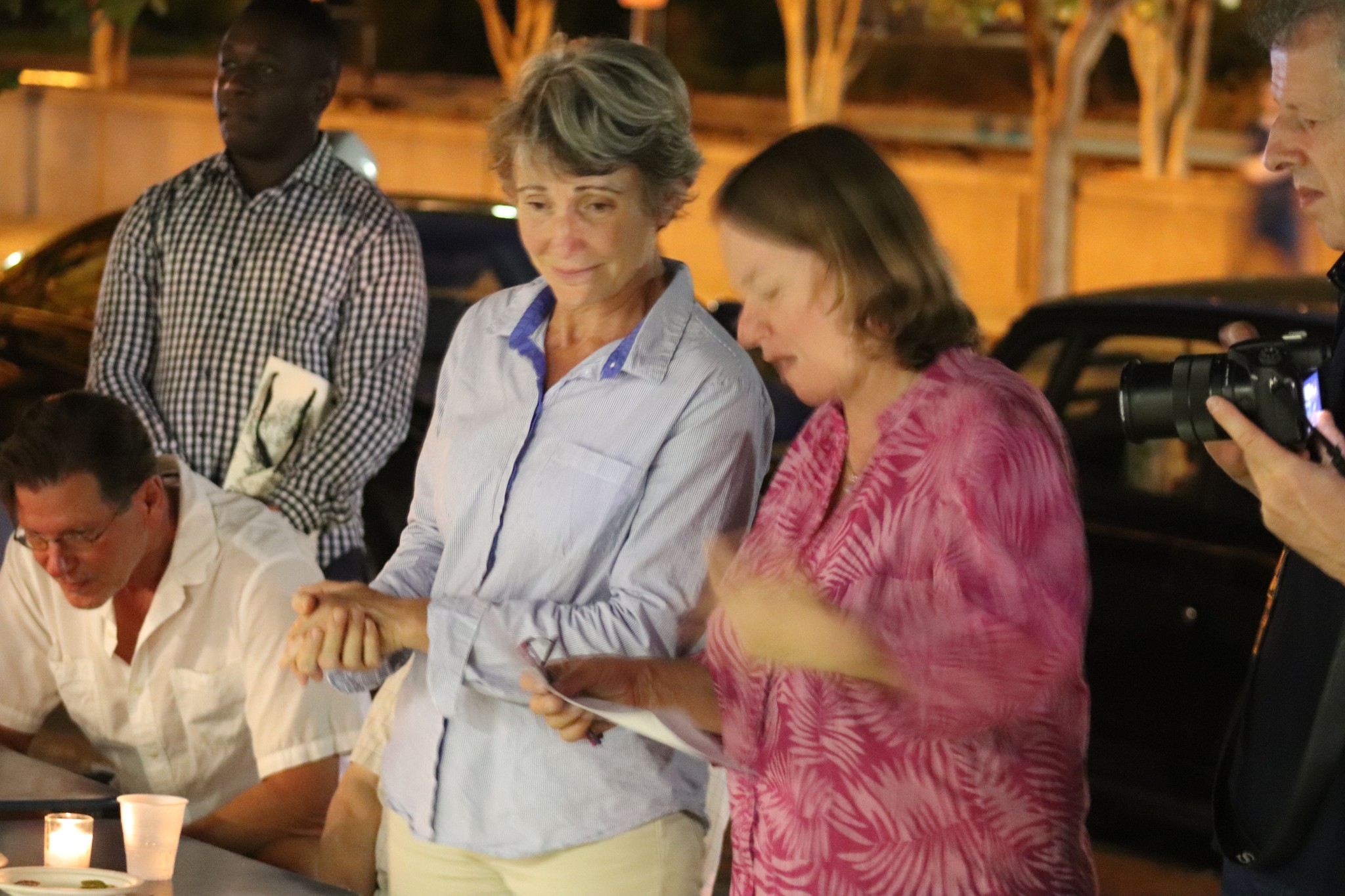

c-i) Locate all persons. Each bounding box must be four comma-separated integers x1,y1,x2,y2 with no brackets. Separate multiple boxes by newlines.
0,393,415,896
285,40,779,896
1194,0,1345,896
82,0,429,583
522,123,1099,896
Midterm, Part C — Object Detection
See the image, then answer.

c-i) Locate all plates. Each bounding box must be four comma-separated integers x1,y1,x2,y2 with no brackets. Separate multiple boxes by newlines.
0,867,145,896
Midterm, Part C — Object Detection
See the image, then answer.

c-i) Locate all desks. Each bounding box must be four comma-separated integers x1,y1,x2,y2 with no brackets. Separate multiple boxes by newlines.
0,745,357,896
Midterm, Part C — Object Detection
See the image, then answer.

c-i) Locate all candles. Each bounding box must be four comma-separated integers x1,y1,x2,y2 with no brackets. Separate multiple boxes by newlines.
41,813,94,868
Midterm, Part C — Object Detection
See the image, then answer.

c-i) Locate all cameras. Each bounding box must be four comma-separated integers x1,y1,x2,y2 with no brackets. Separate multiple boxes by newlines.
1117,330,1334,453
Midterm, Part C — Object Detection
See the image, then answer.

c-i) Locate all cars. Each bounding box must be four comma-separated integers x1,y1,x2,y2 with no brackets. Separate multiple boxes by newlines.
989,273,1345,670
0,186,545,566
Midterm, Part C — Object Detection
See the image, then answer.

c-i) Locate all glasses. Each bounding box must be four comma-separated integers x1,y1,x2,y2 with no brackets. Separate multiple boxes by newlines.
12,486,139,552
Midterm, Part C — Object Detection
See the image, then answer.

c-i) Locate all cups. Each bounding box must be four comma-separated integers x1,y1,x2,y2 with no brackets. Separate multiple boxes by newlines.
117,793,190,882
44,813,94,869
